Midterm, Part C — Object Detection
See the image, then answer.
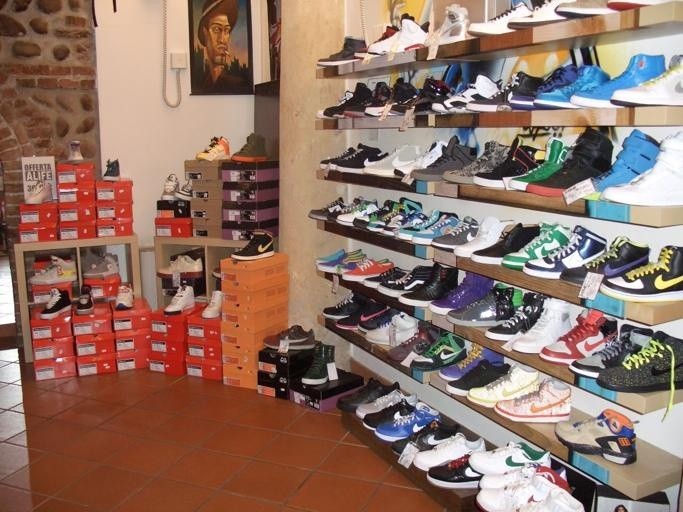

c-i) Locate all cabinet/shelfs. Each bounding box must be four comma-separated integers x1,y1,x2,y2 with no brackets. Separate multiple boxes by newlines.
153,235,250,309
316,0,683,512
14,232,143,364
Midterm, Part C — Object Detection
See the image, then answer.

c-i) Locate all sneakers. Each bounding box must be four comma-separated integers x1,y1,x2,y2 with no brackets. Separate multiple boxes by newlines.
163,285,195,315
24,182,53,205
196,136,230,161
161,173,194,201
116,284,134,310
263,326,315,353
157,255,204,279
317,0,681,206
202,290,223,318
30,255,77,285
83,253,119,279
231,233,275,260
103,159,120,181
302,198,683,512
40,287,72,319
231,134,269,162
76,285,95,315
69,140,83,165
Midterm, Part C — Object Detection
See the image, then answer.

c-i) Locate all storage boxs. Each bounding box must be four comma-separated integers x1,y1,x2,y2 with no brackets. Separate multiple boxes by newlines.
221,255,366,412
18,161,133,242
154,158,279,241
31,297,152,381
149,302,222,380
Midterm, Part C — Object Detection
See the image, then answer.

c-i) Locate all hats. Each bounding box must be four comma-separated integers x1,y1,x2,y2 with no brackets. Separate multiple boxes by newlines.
198,0,238,46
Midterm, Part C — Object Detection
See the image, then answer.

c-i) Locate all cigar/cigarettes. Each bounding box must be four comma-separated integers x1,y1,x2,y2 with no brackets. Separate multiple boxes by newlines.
224,50,231,57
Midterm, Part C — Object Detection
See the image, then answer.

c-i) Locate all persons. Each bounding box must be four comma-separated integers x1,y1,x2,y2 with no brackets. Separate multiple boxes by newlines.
193,0,253,95
